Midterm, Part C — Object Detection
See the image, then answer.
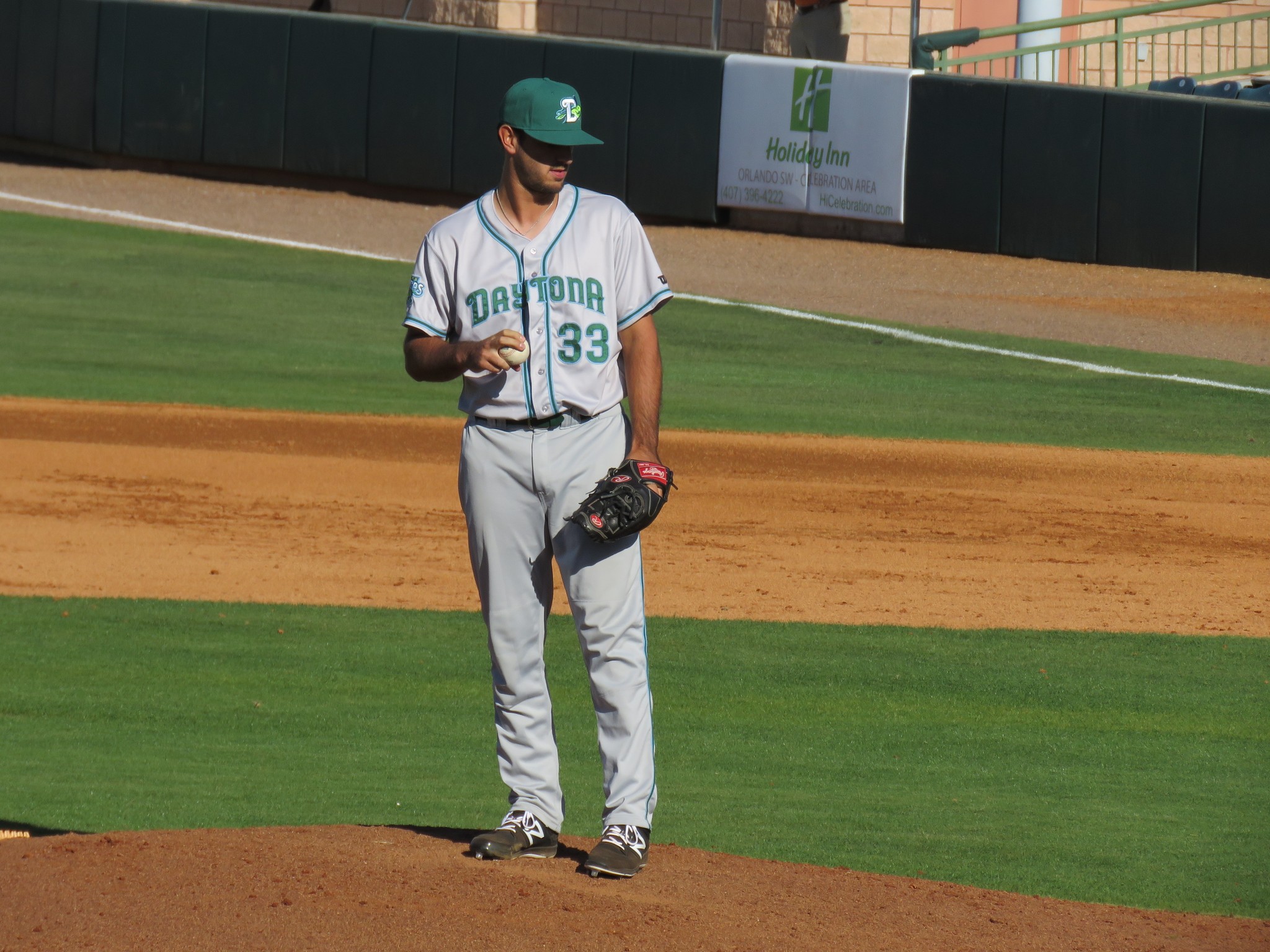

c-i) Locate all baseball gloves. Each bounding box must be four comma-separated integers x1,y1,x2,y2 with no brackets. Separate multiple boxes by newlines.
572,458,674,542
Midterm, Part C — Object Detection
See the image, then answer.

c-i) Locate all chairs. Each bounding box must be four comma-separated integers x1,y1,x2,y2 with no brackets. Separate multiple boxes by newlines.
1148,77,1270,104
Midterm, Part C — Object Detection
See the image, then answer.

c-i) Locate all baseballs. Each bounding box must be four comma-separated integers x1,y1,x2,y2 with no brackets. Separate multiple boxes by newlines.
499,340,532,366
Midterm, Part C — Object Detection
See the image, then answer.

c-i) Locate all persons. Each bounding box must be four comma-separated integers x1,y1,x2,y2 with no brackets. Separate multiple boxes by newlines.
401,78,677,878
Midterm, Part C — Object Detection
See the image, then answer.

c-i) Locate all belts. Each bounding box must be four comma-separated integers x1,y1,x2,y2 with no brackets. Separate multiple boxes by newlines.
475,413,591,429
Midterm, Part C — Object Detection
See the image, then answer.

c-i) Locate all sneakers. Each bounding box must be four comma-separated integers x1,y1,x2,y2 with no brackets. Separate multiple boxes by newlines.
470,809,559,859
584,825,649,876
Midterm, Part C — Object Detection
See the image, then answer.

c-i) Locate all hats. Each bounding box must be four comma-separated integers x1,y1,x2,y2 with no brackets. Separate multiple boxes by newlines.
504,77,605,145
